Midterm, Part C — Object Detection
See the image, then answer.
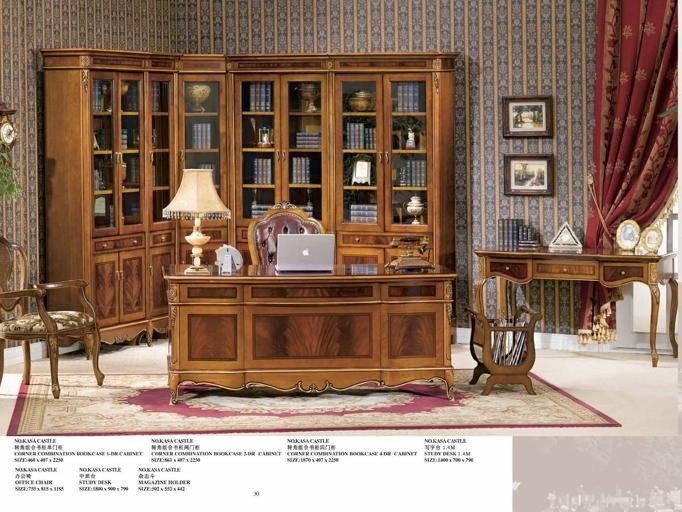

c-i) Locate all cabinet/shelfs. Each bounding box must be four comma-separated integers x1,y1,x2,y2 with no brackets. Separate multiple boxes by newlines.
334,53,456,230
173,55,228,228
174,229,235,271
43,236,173,348
337,231,457,344
467,304,544,398
228,54,333,224
232,227,251,253
40,48,173,236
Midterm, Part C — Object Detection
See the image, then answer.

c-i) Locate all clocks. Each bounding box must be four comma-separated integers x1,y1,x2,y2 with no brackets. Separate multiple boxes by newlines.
0,102,20,172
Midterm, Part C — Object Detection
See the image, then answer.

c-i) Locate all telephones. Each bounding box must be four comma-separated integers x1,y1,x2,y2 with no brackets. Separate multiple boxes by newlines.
384,236,434,272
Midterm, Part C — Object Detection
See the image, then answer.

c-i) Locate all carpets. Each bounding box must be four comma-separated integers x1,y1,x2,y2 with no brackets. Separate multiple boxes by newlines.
7,367,623,438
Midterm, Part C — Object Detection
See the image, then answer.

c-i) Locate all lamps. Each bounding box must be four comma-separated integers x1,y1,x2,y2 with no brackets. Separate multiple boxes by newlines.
162,167,231,275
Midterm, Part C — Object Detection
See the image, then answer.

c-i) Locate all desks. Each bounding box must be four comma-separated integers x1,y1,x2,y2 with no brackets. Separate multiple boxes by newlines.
162,259,460,409
472,246,679,370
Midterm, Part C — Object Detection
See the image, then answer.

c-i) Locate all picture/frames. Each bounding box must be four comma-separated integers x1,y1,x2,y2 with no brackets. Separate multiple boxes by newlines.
350,154,372,186
614,221,641,250
502,96,557,139
641,226,662,253
502,153,557,197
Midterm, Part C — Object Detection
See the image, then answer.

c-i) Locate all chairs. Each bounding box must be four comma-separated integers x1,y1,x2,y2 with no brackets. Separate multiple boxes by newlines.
0,230,105,399
245,202,328,266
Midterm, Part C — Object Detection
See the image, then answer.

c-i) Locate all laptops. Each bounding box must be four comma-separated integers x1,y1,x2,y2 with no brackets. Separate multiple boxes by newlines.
275,233,335,272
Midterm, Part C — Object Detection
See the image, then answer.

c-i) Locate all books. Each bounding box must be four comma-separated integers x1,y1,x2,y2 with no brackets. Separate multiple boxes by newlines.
248,82,426,225
497,217,544,254
92,80,217,186
490,314,529,367
